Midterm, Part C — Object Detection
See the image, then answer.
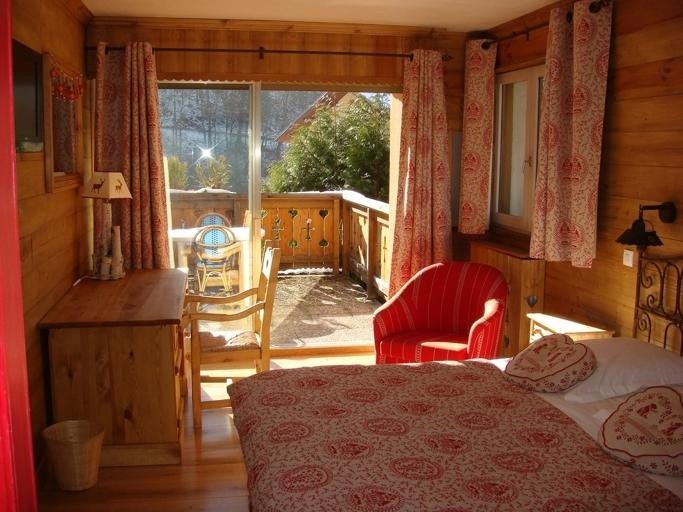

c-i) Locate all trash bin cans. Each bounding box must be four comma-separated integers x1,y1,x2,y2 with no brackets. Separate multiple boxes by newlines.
39,418,106,493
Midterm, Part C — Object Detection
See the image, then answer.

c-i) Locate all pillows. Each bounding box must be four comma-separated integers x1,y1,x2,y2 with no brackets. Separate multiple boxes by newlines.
505,336,683,480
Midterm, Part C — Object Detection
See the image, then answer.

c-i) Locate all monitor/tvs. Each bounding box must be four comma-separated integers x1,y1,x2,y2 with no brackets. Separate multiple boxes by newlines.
12,38,43,152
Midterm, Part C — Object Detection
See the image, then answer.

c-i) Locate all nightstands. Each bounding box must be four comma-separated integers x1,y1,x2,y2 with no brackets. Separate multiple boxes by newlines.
524,310,615,346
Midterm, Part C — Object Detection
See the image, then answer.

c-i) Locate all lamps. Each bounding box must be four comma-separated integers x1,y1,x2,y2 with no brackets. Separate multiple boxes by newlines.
617,202,678,247
80,171,133,279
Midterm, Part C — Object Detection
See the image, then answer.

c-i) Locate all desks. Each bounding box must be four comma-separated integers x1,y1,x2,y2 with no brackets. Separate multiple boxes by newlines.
172,226,266,288
39,268,190,468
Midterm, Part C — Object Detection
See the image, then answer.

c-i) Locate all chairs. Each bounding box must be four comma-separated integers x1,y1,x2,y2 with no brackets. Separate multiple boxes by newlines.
372,260,509,365
183,238,282,430
189,213,236,312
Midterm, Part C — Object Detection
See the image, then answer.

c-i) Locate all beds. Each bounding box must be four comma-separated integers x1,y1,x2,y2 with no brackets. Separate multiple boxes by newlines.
232,248,683,510
